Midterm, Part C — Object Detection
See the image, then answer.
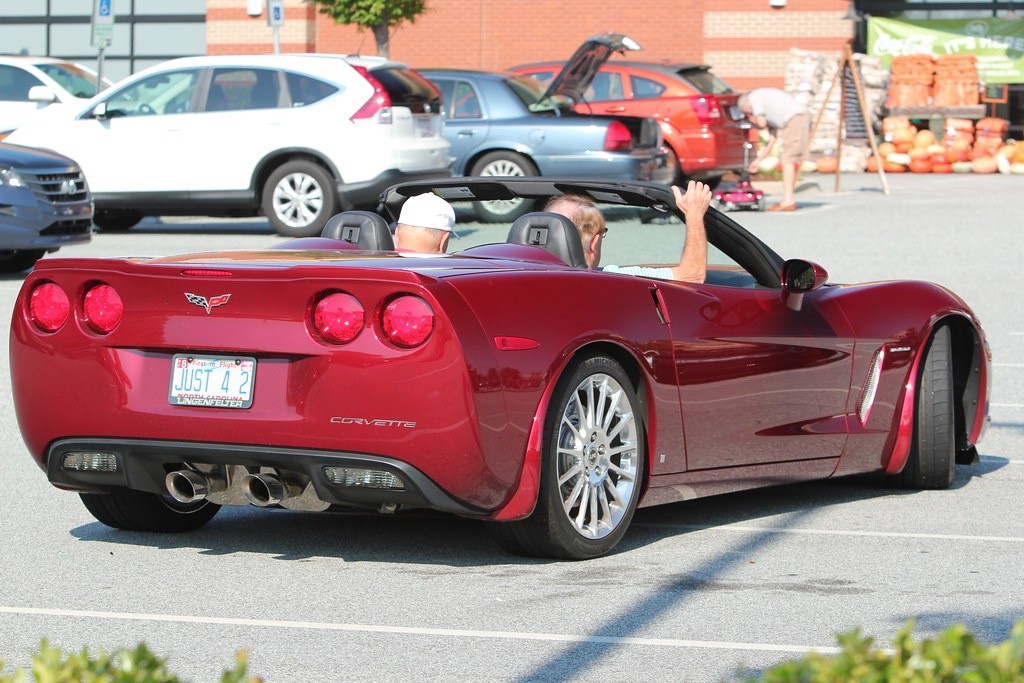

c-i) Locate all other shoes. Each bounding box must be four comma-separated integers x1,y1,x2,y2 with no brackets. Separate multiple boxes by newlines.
769,202,796,211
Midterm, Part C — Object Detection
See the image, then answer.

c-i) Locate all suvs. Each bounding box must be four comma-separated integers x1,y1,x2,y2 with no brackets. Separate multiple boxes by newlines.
506,61,759,191
0,51,459,237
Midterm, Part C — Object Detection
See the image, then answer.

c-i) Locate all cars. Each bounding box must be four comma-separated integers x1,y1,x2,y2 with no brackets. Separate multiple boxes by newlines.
6,171,996,562
414,68,635,224
0,54,159,138
0,141,95,275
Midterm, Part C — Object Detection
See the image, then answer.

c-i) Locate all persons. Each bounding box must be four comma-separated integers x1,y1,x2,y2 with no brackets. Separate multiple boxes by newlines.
737,88,811,211
546,180,712,286
394,192,459,258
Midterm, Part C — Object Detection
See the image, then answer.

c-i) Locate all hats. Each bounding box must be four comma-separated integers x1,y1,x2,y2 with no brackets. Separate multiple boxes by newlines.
397,190,461,240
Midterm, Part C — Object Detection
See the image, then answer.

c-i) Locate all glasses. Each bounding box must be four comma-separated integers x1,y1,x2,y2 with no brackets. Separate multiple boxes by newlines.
591,228,608,242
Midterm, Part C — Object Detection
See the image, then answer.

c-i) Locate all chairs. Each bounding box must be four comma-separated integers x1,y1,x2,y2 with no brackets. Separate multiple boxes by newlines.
506,211,587,268
321,209,397,252
205,85,228,111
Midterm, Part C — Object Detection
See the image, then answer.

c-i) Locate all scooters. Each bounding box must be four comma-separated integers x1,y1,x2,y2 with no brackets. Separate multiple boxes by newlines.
713,119,767,211
